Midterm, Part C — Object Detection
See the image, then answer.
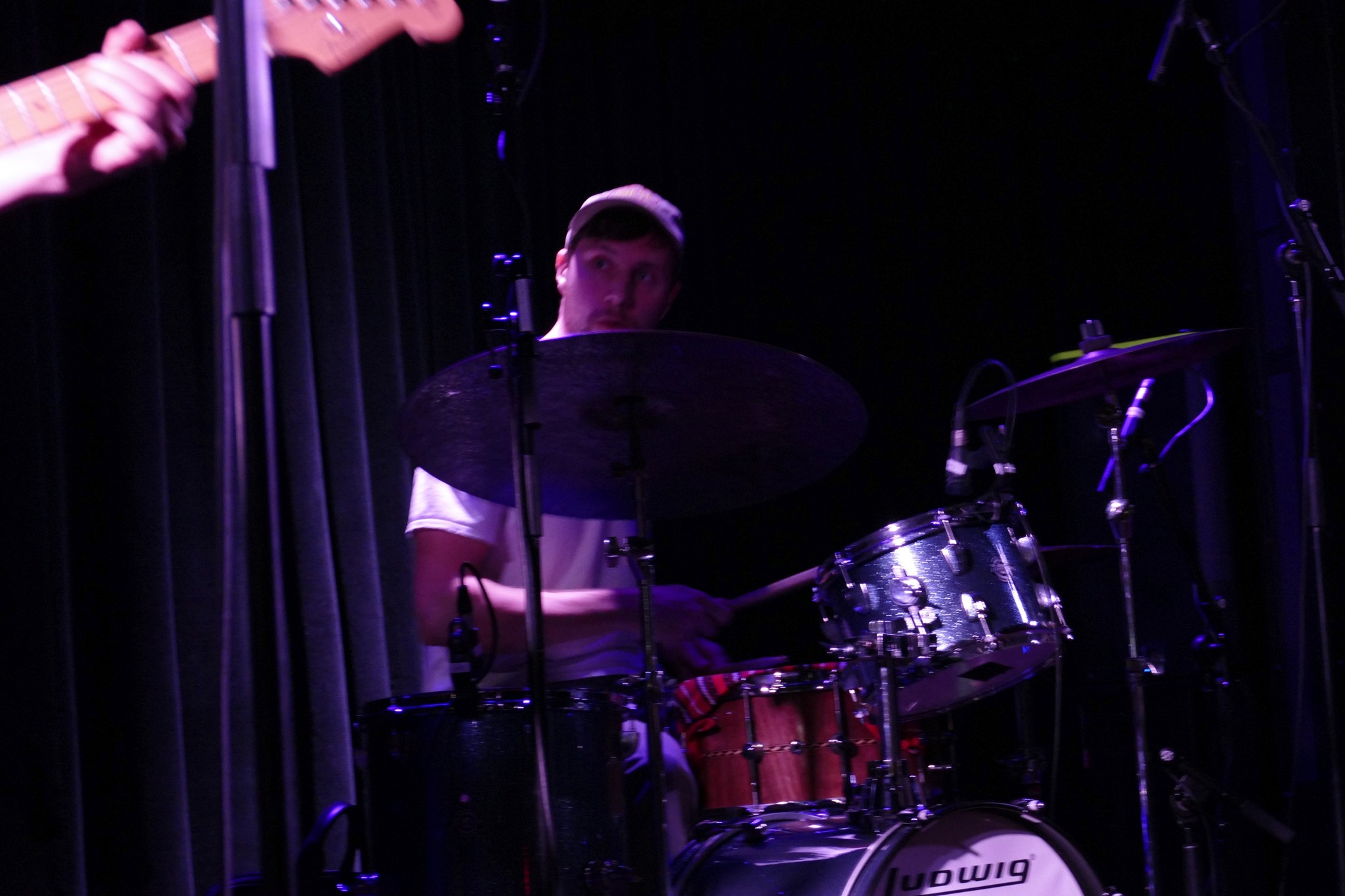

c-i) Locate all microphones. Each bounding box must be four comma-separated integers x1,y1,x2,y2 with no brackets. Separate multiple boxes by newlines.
455,585,489,680
1148,0,1186,83
946,406,968,495
1098,378,1158,495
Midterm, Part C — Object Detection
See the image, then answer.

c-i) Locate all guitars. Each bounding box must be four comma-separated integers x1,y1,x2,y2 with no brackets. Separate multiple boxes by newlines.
0,0,467,153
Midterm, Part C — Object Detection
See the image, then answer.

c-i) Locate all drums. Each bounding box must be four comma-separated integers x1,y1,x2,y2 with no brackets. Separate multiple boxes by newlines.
670,652,934,865
663,800,1108,896
815,497,1073,715
356,674,630,896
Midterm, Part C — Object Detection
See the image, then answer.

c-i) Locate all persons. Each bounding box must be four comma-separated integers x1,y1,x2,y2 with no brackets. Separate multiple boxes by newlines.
406,183,755,896
0,14,203,213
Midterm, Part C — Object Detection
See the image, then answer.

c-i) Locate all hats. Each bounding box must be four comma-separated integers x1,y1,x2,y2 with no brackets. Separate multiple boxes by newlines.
563,184,685,248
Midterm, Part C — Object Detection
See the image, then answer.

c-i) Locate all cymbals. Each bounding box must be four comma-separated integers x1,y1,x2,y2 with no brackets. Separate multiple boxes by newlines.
968,320,1238,420
402,329,862,518
